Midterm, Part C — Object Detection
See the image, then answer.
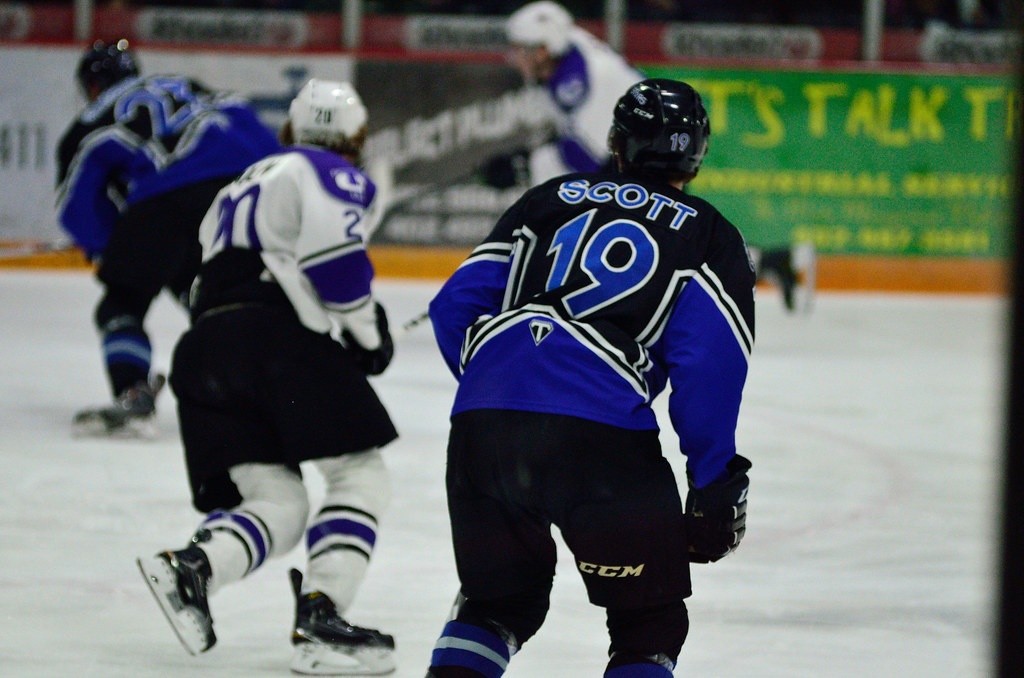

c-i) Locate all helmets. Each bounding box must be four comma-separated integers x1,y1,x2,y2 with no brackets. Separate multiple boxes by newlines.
289,79,369,143
605,78,712,178
503,0,573,58
73,44,138,89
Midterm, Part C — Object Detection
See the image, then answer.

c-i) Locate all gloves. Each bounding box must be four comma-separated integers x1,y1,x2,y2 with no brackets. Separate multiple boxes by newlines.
340,300,394,375
482,146,530,188
681,453,753,563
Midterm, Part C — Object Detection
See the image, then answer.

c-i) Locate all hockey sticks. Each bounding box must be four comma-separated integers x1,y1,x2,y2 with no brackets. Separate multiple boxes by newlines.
388,309,432,343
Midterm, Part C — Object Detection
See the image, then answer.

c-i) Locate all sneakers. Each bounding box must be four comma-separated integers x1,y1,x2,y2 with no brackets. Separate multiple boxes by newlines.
762,238,814,311
135,528,217,657
287,568,397,674
72,374,166,439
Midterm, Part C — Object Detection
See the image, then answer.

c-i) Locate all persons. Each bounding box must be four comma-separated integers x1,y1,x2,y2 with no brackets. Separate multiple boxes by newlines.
427,78,756,678
504,0,647,190
56,41,400,675
